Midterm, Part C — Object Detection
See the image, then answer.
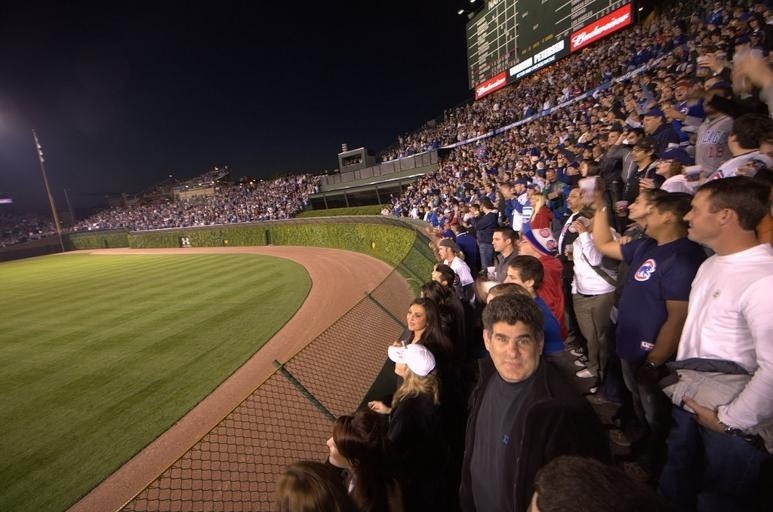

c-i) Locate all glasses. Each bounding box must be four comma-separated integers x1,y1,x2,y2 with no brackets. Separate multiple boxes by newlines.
630,148,644,154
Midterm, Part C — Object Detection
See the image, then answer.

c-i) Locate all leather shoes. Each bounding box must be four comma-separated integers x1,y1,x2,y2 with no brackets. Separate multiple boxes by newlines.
585,393,624,408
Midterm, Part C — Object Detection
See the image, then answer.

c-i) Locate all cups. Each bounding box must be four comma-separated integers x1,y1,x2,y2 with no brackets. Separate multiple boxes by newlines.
616,201,628,218
577,177,596,203
569,215,589,233
487,266,496,279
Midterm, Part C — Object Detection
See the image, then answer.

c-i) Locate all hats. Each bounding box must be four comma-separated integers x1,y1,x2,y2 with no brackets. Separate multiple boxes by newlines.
514,142,595,193
635,136,657,151
653,146,695,165
388,343,436,378
638,109,664,119
522,222,558,258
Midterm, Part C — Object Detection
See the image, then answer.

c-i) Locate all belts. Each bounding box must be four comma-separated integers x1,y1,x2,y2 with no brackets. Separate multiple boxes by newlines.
576,291,598,298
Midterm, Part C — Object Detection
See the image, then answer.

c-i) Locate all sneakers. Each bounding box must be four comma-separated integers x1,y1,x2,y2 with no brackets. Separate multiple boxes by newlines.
575,368,596,379
624,460,655,481
573,358,584,368
570,348,585,358
608,428,636,447
